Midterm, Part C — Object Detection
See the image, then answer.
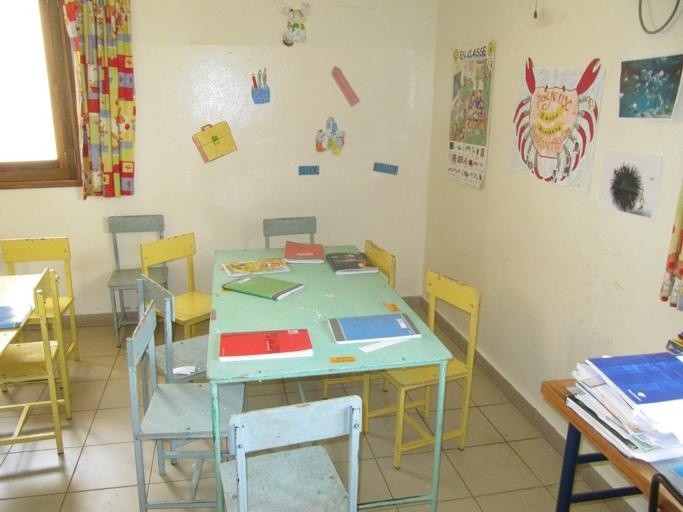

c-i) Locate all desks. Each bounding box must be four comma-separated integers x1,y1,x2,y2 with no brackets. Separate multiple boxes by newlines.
1,273,44,353
205,244,453,511
541,379,683,512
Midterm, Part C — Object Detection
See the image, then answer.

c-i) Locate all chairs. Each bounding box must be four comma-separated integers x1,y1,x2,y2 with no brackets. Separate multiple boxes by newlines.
136,274,209,417
362,268,482,471
219,394,362,512
262,215,317,249
1,267,73,455
106,215,168,349
1,236,80,397
126,298,246,511
321,239,396,398
140,232,213,341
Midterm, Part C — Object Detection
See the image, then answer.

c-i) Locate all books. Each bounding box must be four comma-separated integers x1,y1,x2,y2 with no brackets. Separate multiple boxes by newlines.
220,274,307,302
283,238,325,264
218,255,292,278
325,311,422,347
218,327,314,365
0,303,33,330
324,251,381,277
563,330,682,487
